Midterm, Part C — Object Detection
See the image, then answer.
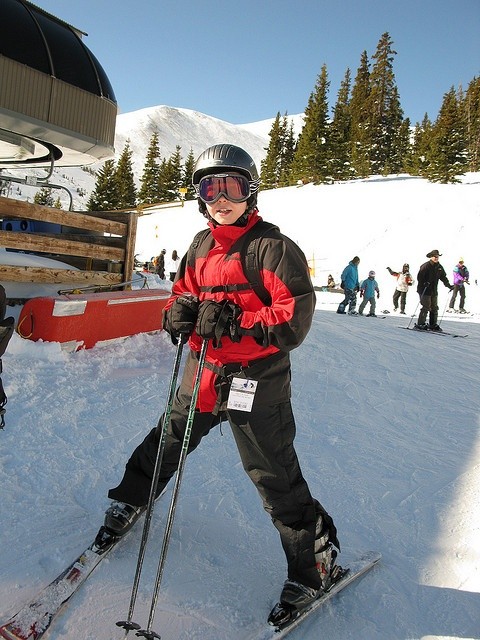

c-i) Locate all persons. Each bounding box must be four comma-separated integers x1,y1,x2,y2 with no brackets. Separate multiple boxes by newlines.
358,271,379,317
169,249,182,280
447,258,470,314
327,274,335,288
103,144,339,609
337,256,361,316
385,262,414,315
416,250,454,332
155,248,166,279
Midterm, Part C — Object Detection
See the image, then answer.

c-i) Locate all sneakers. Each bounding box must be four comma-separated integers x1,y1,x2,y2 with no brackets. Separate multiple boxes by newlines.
105,501,145,535
280,544,334,608
419,323,429,330
429,324,442,331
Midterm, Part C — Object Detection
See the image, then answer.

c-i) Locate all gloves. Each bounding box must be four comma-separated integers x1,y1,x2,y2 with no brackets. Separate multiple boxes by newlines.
195,299,242,343
165,292,199,347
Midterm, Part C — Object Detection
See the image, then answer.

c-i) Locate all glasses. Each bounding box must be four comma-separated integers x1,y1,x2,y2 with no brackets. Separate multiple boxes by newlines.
196,173,250,204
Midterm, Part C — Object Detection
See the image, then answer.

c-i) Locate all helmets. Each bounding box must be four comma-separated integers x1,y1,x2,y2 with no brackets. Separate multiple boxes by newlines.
191,143,258,206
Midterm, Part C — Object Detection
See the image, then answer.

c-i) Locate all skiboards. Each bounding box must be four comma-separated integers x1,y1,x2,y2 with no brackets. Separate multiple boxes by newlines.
0,485,383,640
398,324,469,338
359,314,386,319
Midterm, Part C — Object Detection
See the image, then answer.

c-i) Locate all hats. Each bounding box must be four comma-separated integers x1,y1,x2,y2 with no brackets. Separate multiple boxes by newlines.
426,250,442,258
369,270,375,277
